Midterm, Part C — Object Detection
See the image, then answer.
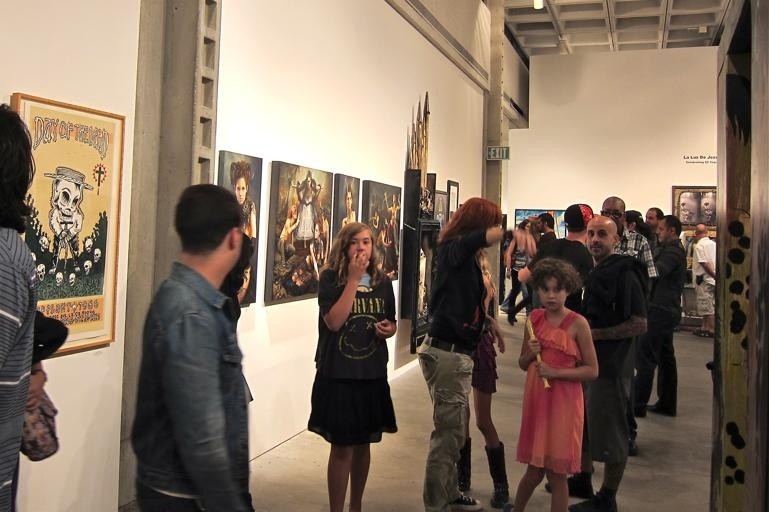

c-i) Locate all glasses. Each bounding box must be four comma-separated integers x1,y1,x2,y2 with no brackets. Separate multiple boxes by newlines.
600,210,624,218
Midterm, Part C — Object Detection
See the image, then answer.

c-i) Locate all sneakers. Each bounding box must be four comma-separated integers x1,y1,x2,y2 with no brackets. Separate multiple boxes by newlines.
569,490,618,512
699,331,715,338
628,439,637,456
448,494,482,511
544,472,594,498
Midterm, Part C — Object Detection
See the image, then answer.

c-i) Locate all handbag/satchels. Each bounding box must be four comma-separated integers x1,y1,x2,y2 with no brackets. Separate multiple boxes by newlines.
505,242,516,267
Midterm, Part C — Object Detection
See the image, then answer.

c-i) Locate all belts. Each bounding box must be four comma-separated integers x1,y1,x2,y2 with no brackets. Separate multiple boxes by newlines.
431,338,474,357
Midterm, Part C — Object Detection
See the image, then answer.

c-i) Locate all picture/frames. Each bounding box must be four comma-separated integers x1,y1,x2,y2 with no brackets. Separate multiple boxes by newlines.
7,93,125,361
446,179,460,227
672,185,717,232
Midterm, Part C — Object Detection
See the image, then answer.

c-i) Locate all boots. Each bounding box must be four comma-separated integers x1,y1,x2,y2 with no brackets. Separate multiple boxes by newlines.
457,438,471,491
484,442,509,507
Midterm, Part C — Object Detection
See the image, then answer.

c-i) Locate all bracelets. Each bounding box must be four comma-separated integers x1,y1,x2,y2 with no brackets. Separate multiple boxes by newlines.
31,368,49,382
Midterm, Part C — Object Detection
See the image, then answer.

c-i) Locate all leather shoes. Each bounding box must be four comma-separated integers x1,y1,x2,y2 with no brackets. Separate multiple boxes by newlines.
647,401,676,417
505,306,515,325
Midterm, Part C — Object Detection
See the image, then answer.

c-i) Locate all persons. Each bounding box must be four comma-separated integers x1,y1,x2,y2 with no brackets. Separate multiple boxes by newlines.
417,196,538,512
546,216,648,512
128,184,252,512
382,191,401,221
536,214,556,247
342,186,355,229
692,224,716,337
0,104,34,509
307,220,400,511
376,224,387,250
638,215,687,416
600,199,658,301
626,211,657,255
645,207,663,229
387,226,399,271
276,207,296,261
228,161,257,237
504,220,532,326
321,208,329,259
457,248,509,506
305,223,324,271
511,260,599,512
517,203,594,282
368,207,380,232
17,348,57,416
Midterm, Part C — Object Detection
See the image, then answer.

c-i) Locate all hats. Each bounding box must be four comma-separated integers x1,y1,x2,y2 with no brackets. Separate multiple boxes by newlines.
564,204,600,228
